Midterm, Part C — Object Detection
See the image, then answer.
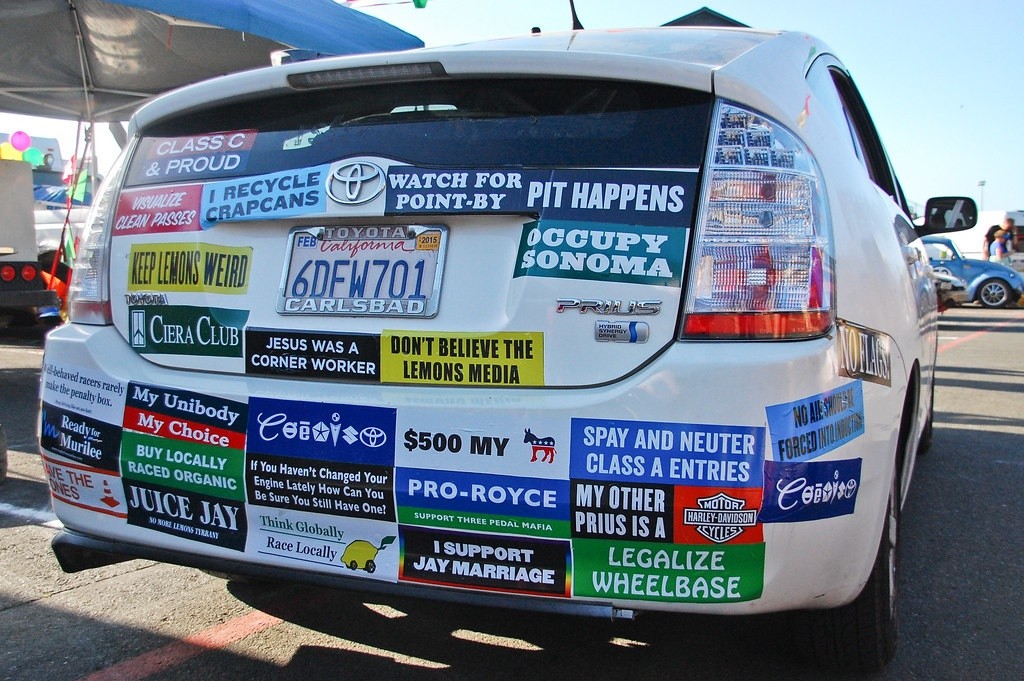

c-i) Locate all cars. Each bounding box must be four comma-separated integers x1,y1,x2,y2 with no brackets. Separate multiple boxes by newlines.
933,271,970,308
34,168,105,331
922,236,1023,308
33,7,979,659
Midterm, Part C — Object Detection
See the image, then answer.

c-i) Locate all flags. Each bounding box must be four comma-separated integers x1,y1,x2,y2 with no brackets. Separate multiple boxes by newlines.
60,153,89,267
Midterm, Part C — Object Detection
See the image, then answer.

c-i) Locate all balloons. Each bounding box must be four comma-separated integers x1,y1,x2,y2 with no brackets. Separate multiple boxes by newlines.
0,131,44,167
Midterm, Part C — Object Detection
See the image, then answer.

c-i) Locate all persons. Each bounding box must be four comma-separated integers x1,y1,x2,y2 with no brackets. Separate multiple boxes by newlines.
983,218,1016,267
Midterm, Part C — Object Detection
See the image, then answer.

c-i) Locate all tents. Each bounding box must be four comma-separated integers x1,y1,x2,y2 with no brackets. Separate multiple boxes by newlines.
0,0,426,203
